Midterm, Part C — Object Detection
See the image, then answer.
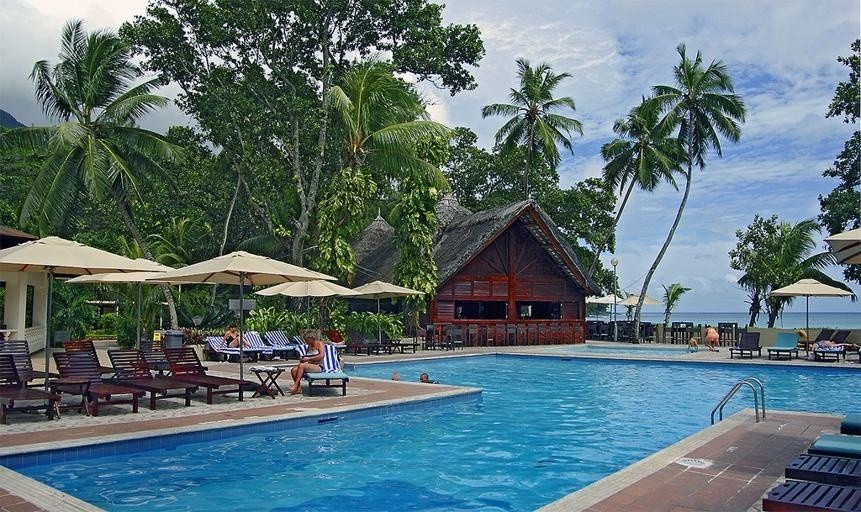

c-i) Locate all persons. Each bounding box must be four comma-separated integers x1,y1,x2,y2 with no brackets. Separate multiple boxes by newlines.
704,326,717,349
809,338,837,355
220,323,252,348
288,330,327,396
420,371,439,384
390,372,401,381
703,326,720,345
792,326,808,342
687,335,699,353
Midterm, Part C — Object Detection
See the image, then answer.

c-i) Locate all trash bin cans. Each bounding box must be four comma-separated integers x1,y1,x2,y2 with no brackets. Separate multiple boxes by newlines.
164,331,184,361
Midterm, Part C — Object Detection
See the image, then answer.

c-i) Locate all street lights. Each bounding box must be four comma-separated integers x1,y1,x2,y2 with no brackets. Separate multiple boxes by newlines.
609,257,620,342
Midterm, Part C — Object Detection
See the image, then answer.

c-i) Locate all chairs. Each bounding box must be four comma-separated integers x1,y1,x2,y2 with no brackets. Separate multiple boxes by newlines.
422,322,583,352
729,328,860,362
759,406,861,512
586,320,749,346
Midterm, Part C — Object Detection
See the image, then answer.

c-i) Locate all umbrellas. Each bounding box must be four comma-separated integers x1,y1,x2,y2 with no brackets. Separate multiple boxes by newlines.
147,244,338,404
589,293,624,322
823,226,861,267
62,253,214,351
618,293,663,305
1,230,163,395
257,276,362,327
349,280,425,341
770,277,853,357
